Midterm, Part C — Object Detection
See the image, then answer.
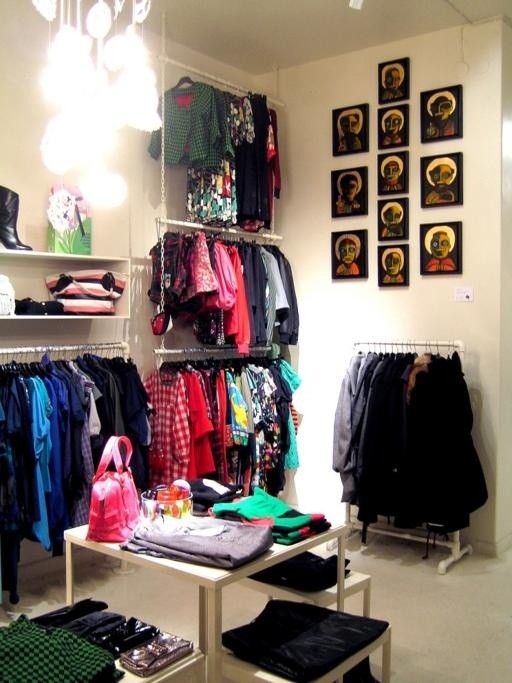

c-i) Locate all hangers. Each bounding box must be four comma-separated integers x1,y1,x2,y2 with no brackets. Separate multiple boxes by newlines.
0,341,126,387
172,68,195,89
157,343,270,377
324,338,476,578
165,217,279,254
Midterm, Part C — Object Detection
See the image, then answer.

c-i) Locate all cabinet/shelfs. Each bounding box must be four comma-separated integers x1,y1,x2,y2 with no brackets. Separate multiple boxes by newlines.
0,598,205,683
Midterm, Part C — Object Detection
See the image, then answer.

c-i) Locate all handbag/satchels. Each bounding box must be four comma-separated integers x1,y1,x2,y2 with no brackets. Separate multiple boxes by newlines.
150,217,173,336
45,269,129,315
84,436,140,544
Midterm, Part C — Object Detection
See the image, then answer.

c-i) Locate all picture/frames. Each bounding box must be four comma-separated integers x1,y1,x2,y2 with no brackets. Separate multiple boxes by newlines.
419,84,464,144
377,103,410,150
377,56,411,105
377,197,410,242
332,102,369,156
376,150,409,196
377,244,410,288
331,229,368,281
330,166,369,219
419,221,463,276
419,152,466,208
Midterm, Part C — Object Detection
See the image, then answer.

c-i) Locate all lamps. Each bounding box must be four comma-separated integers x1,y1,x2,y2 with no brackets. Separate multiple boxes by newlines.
29,1,163,216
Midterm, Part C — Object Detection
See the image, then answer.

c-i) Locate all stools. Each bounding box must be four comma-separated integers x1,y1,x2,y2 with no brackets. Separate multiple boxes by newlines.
236,569,372,618
222,599,392,683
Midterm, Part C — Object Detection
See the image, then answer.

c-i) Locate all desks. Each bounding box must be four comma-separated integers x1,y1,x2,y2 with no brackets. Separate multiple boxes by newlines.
62,522,350,683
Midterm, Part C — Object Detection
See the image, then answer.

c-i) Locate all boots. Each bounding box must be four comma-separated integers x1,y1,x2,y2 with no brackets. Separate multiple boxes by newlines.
0,184,33,252
